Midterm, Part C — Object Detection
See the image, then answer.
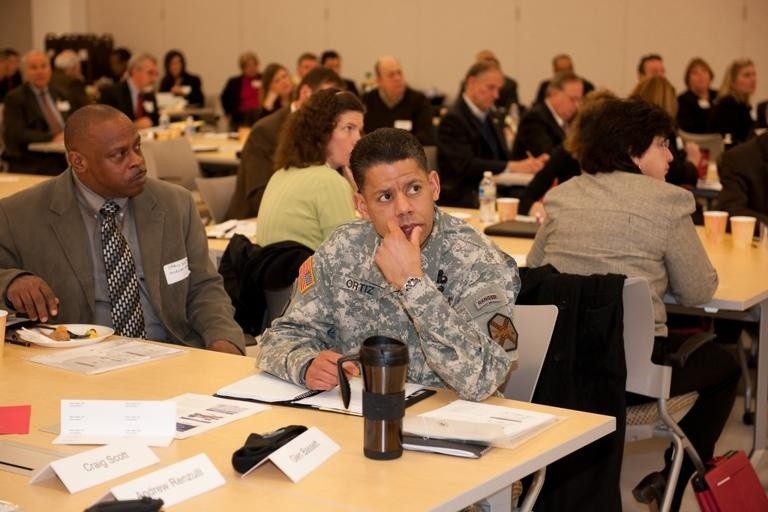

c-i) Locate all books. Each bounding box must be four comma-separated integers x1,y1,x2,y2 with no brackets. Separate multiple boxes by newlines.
212,364,441,419
398,434,496,461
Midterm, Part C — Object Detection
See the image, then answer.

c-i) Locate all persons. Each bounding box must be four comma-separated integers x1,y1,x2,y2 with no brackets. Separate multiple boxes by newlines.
0,44,205,175
221,67,347,222
244,127,524,510
531,94,744,512
508,54,768,373
218,50,550,209
0,103,247,356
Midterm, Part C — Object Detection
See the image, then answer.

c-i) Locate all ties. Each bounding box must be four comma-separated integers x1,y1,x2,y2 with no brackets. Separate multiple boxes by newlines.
98,199,147,342
135,91,144,120
39,89,63,137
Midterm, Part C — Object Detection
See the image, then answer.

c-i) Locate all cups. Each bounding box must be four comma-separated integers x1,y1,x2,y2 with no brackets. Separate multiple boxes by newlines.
695,149,710,181
337,337,410,460
159,115,171,129
703,210,729,247
238,128,251,147
728,215,758,253
497,197,520,224
0,309,8,360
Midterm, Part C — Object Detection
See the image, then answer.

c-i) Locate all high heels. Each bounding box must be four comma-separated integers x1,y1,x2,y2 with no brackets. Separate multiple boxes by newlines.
631,469,683,512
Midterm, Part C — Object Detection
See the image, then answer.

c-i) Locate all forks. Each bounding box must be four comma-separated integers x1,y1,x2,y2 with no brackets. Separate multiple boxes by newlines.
32,324,90,339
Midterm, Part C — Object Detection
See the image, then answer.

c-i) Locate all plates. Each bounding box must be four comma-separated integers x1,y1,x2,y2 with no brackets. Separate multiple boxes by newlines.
19,323,115,348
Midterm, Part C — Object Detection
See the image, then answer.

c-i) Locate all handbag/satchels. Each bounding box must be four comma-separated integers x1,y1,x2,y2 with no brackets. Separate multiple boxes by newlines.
690,448,767,512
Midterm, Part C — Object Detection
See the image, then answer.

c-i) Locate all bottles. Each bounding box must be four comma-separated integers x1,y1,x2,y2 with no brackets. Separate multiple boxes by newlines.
479,171,496,224
184,115,196,144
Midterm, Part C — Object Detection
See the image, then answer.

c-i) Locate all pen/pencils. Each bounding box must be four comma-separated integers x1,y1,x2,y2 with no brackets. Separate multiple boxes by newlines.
526,150,534,158
224,225,236,232
5,339,30,346
